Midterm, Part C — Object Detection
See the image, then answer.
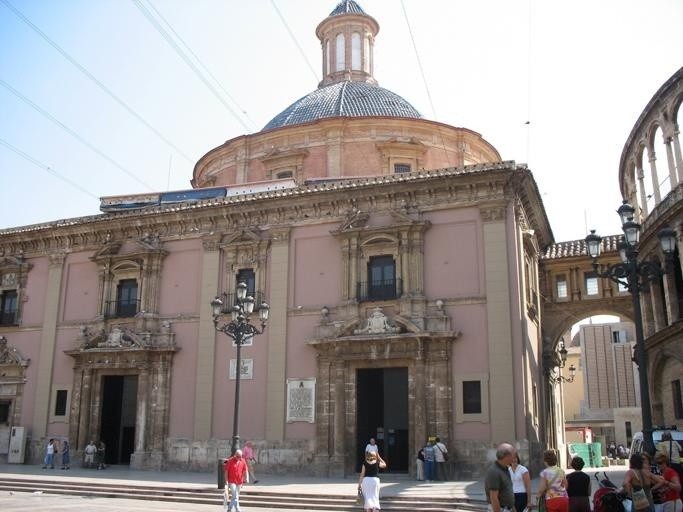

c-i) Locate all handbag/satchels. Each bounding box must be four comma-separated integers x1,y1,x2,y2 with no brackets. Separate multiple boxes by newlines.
356,489,365,505
632,488,649,510
442,452,449,460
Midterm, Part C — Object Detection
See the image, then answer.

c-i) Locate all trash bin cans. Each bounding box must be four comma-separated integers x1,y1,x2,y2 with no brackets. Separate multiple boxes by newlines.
217,458,229,489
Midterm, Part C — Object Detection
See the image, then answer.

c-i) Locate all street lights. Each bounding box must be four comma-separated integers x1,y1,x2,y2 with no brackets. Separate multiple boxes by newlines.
584,198,677,462
210,282,270,459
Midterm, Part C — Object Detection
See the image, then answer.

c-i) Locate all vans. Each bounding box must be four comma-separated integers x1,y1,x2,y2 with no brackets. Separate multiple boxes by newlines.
627,425,682,473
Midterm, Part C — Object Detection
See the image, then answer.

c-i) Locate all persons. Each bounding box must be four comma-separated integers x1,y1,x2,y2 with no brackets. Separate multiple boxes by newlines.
605,441,629,459
508,451,531,511
638,451,664,489
622,453,664,511
96,441,106,469
358,451,386,511
536,450,569,512
41,438,57,468
415,446,425,480
83,440,96,468
364,438,384,473
424,442,434,481
484,442,516,511
566,456,591,512
224,449,249,511
58,440,69,469
242,440,260,484
653,450,683,511
655,431,682,472
433,437,448,481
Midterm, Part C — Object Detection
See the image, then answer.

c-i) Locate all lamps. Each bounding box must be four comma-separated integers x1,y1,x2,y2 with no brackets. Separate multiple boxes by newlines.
558,336,567,369
554,364,576,389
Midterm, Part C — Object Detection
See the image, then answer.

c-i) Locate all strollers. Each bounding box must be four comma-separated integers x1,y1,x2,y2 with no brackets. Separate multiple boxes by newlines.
592,487,622,512
593,472,632,512
